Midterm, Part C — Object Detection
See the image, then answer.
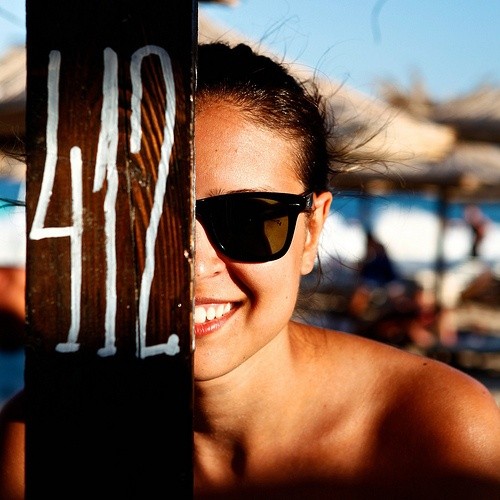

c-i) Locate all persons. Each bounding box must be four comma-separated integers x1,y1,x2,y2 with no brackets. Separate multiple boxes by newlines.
1,41,500,500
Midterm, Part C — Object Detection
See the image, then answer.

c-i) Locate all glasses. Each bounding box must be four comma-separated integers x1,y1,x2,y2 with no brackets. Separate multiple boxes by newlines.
195,188,319,262
